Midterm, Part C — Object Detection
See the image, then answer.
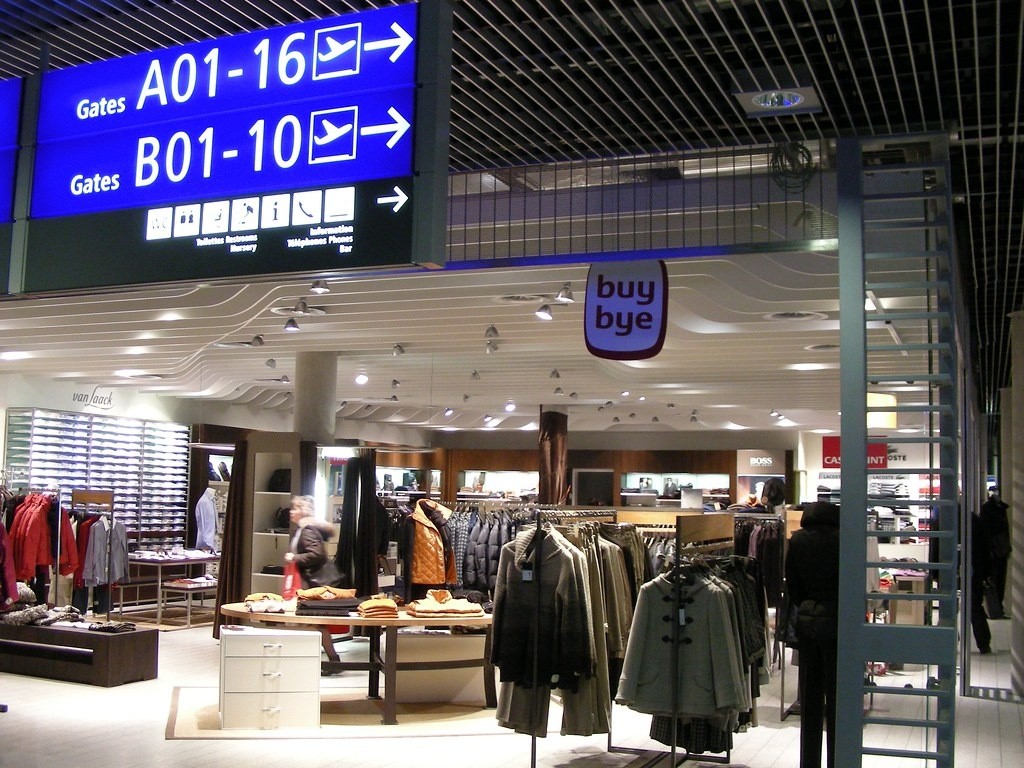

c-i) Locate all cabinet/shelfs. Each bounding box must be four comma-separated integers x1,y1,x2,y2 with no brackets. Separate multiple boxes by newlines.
1,406,193,551
455,471,540,504
205,454,235,588
817,473,942,545
250,453,283,619
375,490,442,511
218,623,323,730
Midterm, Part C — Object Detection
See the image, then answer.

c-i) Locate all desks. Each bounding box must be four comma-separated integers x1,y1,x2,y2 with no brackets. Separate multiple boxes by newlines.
221,603,497,725
119,551,221,625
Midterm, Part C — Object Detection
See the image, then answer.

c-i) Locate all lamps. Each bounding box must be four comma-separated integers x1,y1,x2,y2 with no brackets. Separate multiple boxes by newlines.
310,280,330,295
292,297,310,314
393,343,405,356
284,319,300,331
554,281,575,302
486,340,498,354
484,324,500,339
866,392,897,430
535,304,553,320
251,334,265,346
753,91,804,108
279,368,785,424
265,359,275,369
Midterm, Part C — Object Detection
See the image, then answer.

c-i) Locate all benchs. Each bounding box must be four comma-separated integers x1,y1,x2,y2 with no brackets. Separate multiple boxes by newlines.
162,586,218,626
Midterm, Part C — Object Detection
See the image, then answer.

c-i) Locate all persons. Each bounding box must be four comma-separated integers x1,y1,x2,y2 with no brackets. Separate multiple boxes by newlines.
779,501,841,768
980,495,1010,620
195,487,219,557
929,502,993,655
285,494,346,677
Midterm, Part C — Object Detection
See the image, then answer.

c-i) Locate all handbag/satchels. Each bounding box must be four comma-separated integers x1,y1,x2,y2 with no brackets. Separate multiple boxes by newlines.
282,561,302,601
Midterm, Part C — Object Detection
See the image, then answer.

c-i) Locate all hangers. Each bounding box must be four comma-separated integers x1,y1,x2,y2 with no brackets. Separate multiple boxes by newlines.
0,484,119,521
389,499,782,586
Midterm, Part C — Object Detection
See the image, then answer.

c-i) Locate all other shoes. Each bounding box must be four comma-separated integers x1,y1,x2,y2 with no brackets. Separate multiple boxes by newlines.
991,614,1010,619
321,655,344,675
979,645,991,654
